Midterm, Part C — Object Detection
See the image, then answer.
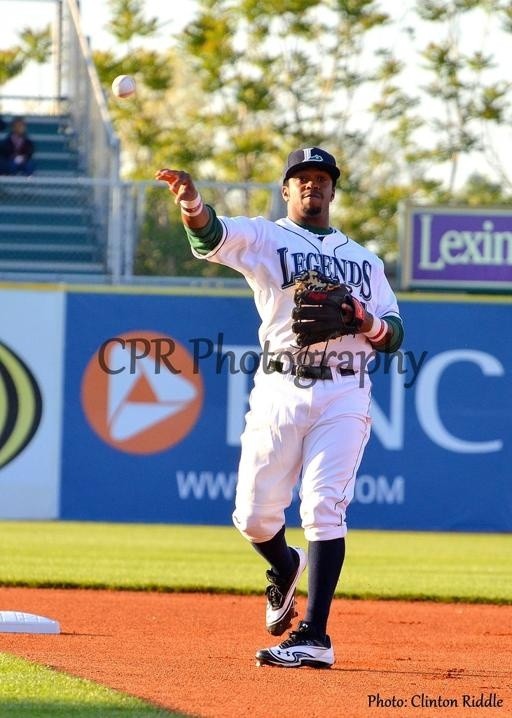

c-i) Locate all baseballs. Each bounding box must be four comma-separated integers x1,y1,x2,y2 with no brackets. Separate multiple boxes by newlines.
114,74,130,98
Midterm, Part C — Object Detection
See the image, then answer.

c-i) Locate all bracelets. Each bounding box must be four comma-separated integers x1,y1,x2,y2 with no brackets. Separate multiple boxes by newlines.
362,313,389,344
179,192,204,218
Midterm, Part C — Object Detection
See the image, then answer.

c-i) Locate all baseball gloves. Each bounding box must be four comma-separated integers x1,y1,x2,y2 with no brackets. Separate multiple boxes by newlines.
291,269,366,348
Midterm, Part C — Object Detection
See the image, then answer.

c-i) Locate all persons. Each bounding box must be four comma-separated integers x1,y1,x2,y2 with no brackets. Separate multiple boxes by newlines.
2,115,36,176
153,146,405,671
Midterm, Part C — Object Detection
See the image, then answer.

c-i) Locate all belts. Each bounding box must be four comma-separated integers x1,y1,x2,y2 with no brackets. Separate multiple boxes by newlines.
267,361,356,378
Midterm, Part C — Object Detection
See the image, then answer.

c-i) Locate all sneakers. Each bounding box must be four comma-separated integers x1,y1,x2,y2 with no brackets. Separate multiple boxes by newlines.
266,546,307,636
255,620,335,668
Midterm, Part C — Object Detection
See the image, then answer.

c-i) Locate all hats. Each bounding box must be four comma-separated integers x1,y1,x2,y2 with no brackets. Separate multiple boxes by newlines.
283,147,341,182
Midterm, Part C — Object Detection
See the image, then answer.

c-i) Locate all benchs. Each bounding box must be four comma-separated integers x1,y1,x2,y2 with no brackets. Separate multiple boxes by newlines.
0,112,106,274
